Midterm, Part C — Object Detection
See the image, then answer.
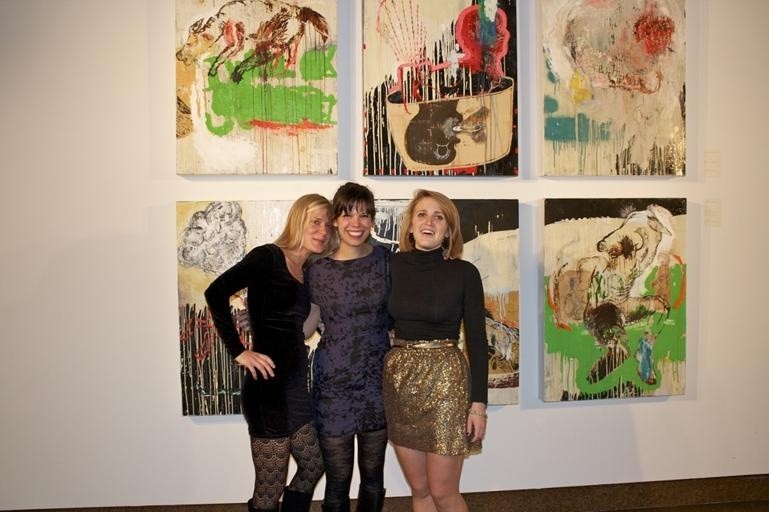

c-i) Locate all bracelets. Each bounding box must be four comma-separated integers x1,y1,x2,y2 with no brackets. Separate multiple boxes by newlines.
468,408,488,419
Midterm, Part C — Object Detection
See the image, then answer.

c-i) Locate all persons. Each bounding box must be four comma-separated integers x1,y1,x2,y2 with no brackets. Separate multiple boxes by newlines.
303,183,392,511
382,188,489,512
205,194,339,511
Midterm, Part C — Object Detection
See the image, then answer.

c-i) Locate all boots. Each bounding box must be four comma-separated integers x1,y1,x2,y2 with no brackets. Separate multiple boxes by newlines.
321,484,386,512
248,486,313,512
